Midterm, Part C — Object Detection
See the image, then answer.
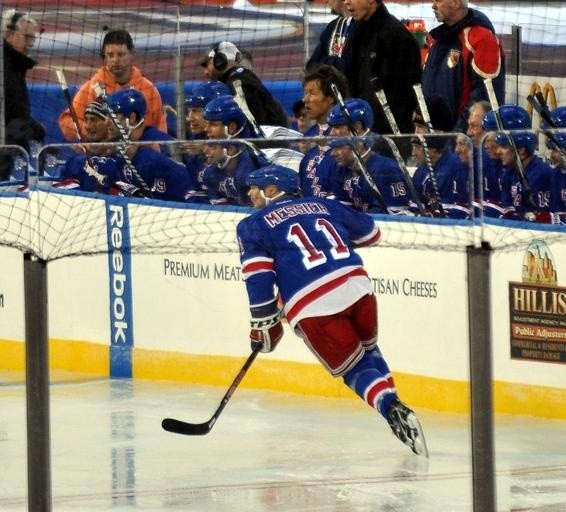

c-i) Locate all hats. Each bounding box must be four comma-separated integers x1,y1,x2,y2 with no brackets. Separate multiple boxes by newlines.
197,39,245,69
409,130,452,153
411,94,460,132
80,94,110,121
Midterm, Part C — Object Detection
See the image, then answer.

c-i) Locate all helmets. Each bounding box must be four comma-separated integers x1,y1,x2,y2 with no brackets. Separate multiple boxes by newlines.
480,104,533,132
103,87,149,124
539,104,566,133
544,129,566,152
493,127,540,157
182,79,234,112
201,91,247,130
243,163,303,197
323,95,378,133
326,132,382,151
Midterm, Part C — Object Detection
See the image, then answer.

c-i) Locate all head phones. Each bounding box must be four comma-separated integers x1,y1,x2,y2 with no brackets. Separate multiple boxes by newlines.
213,41,228,71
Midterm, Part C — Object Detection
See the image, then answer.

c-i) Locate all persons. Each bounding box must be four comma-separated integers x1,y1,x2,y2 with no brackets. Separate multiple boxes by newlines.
237,165,422,455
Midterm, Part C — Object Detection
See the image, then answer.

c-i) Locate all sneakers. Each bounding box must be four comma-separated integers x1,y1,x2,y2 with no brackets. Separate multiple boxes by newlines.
386,396,421,457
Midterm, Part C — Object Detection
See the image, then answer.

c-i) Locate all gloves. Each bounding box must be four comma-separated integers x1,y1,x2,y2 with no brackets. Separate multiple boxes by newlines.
245,296,288,356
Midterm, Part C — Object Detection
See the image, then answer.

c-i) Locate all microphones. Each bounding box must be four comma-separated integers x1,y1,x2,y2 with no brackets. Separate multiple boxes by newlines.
98,25,108,51
34,29,45,40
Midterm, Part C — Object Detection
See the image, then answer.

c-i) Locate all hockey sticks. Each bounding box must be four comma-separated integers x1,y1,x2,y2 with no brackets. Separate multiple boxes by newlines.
162,311,286,435
